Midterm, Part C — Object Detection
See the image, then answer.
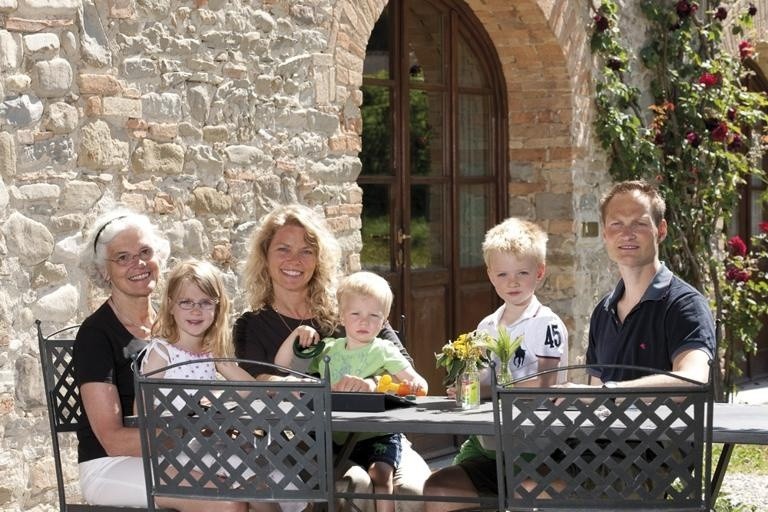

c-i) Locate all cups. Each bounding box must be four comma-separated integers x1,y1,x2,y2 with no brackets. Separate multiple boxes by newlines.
535,354,560,386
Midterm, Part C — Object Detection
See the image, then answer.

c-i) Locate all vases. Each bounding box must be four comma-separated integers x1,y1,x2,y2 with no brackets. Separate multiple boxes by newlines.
455,360,481,410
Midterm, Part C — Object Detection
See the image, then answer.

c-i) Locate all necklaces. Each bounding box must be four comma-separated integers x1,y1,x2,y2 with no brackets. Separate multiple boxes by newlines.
272,302,310,333
109,297,160,335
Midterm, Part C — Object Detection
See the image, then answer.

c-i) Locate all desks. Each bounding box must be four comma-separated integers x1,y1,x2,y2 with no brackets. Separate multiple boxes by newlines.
124,396,768,512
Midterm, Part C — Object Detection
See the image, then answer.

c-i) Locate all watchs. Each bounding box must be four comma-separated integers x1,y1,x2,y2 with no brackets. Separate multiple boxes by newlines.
601,381,619,402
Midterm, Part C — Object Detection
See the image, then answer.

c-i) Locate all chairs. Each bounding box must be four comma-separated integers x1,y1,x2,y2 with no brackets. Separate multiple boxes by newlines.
130,353,335,512
35,320,148,512
490,359,714,512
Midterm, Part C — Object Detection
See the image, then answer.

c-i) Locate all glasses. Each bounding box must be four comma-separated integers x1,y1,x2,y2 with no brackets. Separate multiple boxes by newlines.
172,297,220,311
103,249,154,266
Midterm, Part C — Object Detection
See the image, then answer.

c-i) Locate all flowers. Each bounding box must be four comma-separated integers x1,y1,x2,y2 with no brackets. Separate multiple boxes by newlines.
434,329,489,385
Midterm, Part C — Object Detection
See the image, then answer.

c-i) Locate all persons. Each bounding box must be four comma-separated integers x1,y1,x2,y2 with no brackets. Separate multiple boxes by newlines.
506,178,719,511
230,204,434,511
273,271,429,511
130,255,288,511
74,209,310,511
420,215,570,511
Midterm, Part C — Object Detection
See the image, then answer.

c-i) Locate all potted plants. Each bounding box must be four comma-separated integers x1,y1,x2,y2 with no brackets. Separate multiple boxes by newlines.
489,325,525,411
